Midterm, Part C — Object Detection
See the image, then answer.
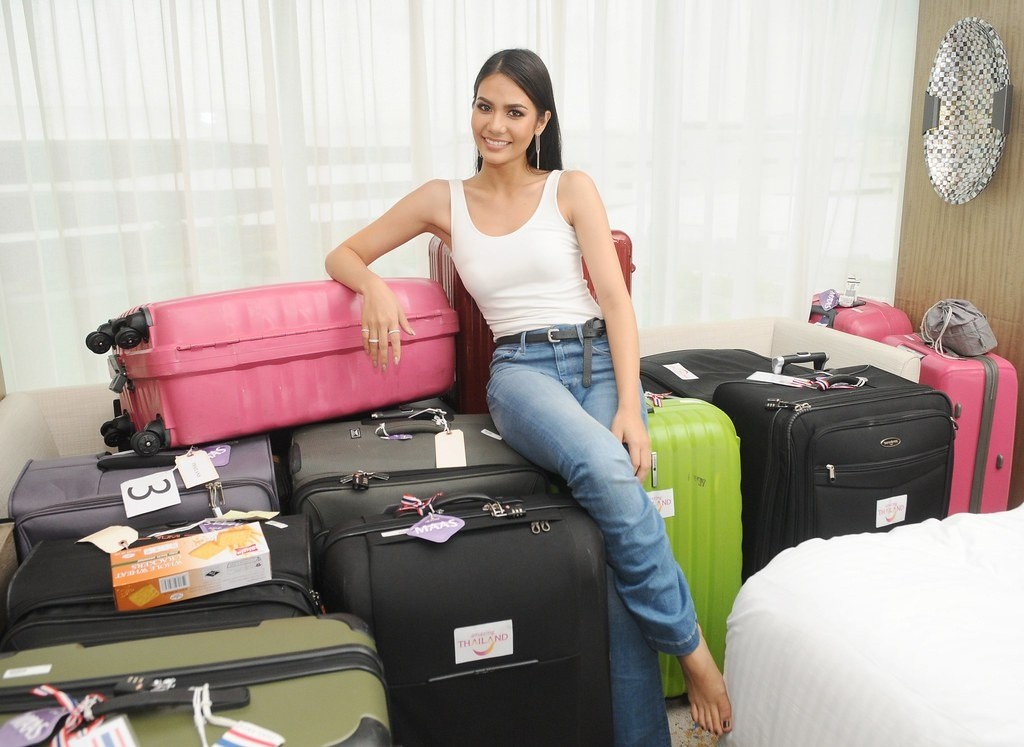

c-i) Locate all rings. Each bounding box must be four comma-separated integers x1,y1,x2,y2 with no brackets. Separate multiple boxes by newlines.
389,330,400,335
368,339,379,342
361,330,369,332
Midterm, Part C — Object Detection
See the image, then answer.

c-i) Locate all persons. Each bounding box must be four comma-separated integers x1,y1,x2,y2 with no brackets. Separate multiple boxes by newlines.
324,49,732,747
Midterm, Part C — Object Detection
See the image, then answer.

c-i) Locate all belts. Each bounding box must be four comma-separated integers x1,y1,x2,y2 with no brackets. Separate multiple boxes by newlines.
497,316,607,388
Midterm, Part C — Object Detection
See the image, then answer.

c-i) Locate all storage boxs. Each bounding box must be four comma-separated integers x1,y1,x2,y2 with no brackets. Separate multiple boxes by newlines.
110,521,272,612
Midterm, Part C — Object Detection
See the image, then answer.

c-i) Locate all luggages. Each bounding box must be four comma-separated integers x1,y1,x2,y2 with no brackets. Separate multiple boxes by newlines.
85,276,462,460
428,229,637,411
0,289,1019,747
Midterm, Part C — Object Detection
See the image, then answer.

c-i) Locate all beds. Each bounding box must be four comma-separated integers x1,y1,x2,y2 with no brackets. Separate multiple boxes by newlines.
726,504,1024,747
